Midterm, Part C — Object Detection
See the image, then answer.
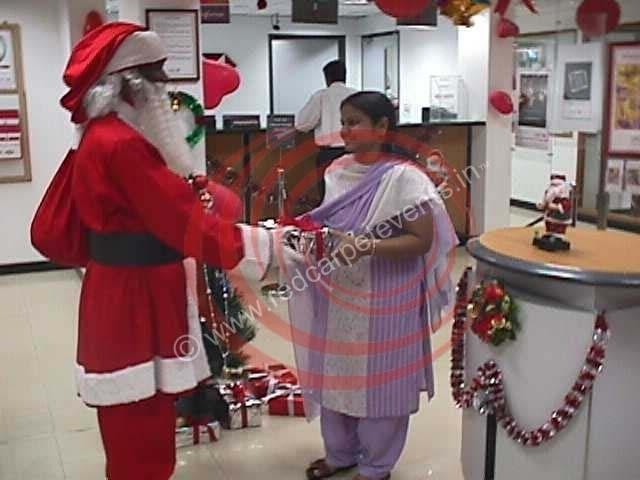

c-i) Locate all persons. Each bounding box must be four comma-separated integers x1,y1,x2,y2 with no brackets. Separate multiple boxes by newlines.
28,20,311,480
531,172,573,252
274,92,459,480
293,58,355,203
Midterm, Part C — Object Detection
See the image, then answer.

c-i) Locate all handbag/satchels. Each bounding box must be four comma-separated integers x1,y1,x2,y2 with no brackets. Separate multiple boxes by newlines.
30,148,89,267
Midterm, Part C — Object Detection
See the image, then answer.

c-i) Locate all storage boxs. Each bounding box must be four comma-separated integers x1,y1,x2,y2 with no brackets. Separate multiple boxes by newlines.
171,360,319,452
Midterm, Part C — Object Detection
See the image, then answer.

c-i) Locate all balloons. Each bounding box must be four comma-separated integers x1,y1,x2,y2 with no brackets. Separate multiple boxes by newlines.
203,57,242,109
575,0,622,43
491,90,514,116
495,17,520,38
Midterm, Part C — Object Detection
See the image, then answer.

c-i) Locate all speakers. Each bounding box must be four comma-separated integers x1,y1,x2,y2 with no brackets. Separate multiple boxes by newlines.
292,1,338,23
397,1,437,26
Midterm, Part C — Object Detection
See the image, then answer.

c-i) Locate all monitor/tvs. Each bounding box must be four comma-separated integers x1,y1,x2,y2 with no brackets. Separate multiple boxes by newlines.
204,116,216,133
422,107,456,122
223,115,260,130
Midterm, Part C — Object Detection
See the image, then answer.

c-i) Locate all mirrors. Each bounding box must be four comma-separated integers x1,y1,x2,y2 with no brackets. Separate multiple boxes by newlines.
360,29,400,121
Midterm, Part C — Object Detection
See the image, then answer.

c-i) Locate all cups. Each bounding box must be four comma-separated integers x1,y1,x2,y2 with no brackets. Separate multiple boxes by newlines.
422,107,430,122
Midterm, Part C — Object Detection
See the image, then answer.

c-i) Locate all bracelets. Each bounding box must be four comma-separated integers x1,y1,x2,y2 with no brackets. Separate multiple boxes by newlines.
372,239,377,256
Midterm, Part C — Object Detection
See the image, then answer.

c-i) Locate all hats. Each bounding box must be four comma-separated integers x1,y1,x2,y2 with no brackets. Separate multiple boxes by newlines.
59,20,167,126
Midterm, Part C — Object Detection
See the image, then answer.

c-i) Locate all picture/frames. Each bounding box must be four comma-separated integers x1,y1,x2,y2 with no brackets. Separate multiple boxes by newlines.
145,4,201,83
607,40,638,158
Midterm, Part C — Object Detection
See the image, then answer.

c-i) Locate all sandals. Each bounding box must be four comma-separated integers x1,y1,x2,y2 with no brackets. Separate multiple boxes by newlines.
306,459,360,479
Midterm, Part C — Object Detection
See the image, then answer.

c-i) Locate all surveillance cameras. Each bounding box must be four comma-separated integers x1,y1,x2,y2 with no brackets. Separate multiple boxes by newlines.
271,12,281,30
